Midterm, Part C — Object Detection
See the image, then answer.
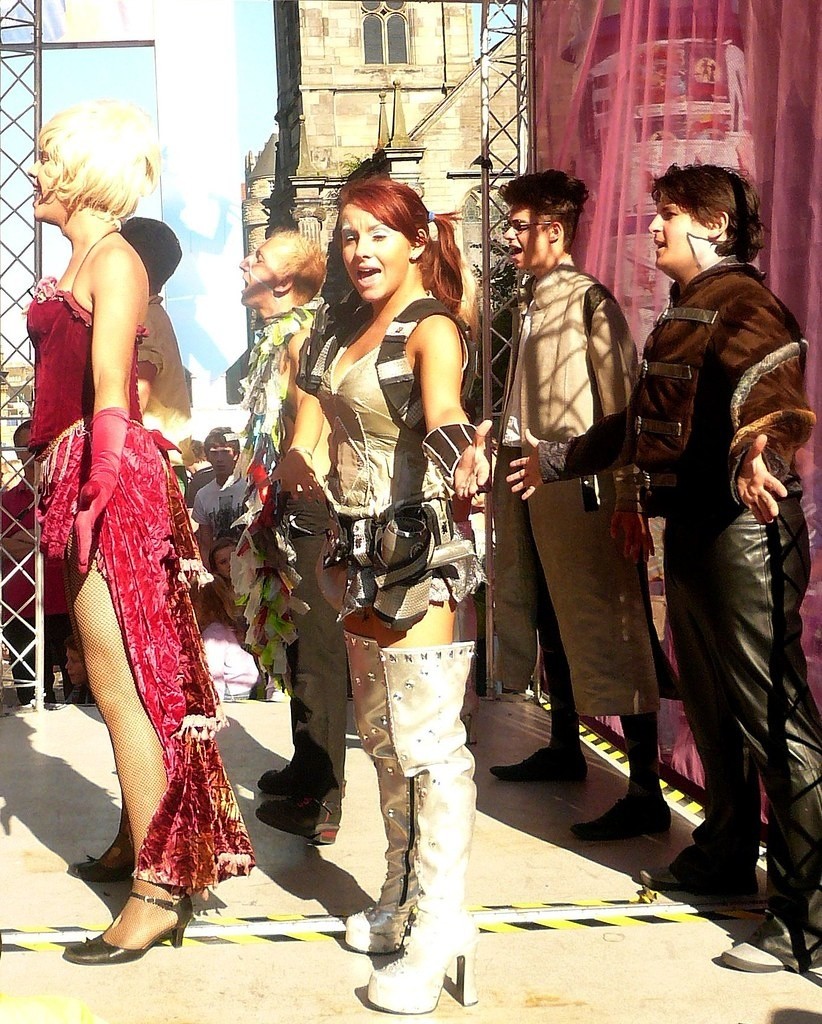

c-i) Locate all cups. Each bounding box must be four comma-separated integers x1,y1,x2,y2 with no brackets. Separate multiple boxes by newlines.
381,517,426,565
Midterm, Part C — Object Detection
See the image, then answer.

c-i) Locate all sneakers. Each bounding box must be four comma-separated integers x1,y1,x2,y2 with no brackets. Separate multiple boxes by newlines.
570,792,671,841
721,942,790,972
258,760,346,798
489,748,588,782
640,867,686,892
256,794,340,843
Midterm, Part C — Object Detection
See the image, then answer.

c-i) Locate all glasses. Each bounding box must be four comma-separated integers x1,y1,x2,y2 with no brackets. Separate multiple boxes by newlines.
502,219,552,235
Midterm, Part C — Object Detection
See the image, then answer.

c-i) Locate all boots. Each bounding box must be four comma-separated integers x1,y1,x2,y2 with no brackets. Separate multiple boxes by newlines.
341,629,420,955
367,641,480,1015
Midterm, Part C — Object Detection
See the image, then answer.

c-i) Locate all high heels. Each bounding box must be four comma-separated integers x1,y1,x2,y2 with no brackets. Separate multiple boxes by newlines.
64,891,193,964
68,830,134,882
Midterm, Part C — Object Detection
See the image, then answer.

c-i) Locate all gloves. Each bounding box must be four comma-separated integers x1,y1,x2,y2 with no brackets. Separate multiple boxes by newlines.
263,446,326,503
73,407,131,574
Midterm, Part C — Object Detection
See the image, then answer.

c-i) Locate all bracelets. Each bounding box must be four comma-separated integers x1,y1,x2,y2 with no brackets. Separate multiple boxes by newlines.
287,447,312,463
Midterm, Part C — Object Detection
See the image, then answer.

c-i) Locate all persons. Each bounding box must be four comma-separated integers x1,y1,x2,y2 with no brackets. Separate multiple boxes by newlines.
313,178,494,1017
182,427,292,702
0,418,99,707
60,635,95,707
505,163,822,975
121,217,191,504
24,98,255,967
227,228,349,847
480,171,686,844
